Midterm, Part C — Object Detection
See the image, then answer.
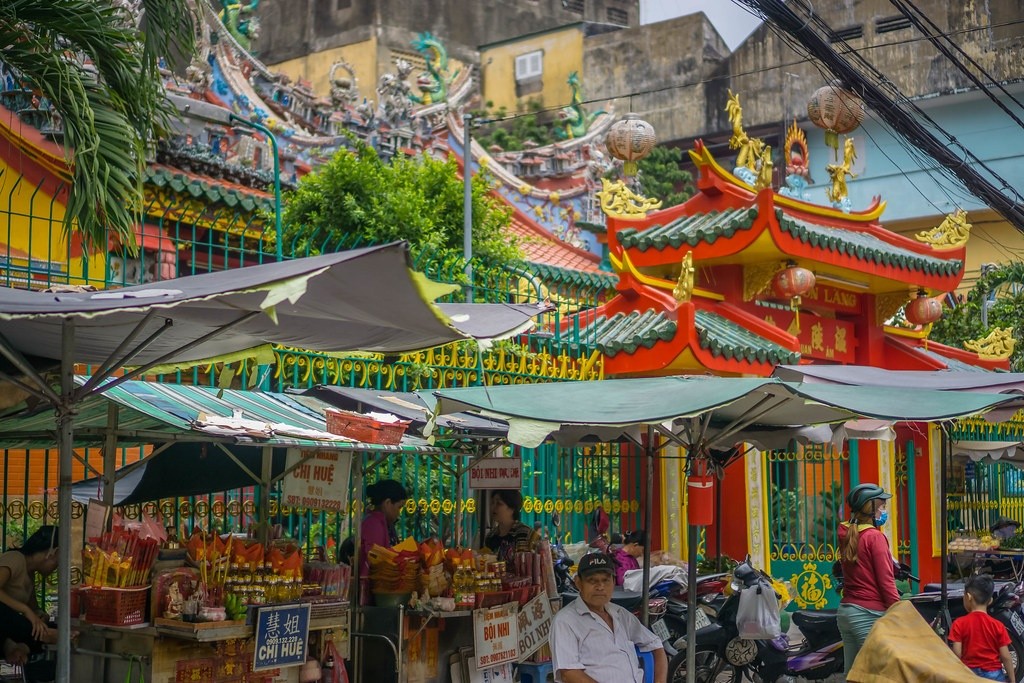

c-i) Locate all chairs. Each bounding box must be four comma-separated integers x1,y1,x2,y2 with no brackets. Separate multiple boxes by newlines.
634,647,655,683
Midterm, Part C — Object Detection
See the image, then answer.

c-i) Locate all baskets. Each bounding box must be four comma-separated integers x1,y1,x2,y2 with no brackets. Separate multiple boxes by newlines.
80,583,150,626
324,408,409,445
300,596,351,618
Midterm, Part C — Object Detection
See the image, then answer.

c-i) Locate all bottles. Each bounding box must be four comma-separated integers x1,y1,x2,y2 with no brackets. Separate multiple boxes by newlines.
222,561,322,619
309,627,349,656
451,545,513,611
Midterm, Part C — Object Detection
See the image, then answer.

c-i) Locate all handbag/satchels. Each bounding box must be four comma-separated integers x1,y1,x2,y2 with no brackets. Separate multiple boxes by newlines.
735,583,781,640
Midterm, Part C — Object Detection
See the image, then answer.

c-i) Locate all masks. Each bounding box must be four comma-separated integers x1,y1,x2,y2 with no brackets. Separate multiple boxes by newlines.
874,509,887,526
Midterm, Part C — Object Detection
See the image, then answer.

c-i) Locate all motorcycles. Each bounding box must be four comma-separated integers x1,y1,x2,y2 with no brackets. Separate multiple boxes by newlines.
532,514,742,683
833,511,1024,683
665,554,844,683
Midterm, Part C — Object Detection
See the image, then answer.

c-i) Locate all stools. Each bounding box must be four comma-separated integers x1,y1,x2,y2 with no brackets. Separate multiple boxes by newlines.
514,661,554,683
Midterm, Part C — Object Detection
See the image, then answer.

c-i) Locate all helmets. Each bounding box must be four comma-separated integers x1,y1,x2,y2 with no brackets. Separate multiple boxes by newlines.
846,483,893,512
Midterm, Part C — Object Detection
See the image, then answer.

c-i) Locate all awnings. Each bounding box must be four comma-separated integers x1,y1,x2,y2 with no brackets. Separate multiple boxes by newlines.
0,373,509,541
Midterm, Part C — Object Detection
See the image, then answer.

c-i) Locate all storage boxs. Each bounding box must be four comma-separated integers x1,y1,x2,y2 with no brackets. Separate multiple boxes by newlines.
299,602,349,618
322,412,410,446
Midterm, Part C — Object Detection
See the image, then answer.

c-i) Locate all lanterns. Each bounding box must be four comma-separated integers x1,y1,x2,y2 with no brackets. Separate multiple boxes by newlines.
606,112,656,176
771,261,815,333
905,290,943,351
807,78,864,160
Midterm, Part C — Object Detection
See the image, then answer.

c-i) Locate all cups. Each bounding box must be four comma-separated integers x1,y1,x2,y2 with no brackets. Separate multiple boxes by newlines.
182,601,197,623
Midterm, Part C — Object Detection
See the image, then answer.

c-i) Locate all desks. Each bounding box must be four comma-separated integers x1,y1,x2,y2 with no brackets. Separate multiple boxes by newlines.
949,549,1024,590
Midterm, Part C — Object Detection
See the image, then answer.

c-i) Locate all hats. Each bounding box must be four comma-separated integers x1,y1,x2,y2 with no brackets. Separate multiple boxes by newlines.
578,553,613,575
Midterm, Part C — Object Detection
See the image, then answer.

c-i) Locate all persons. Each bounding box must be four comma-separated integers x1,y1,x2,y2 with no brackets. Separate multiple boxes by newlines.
982,516,1024,577
610,530,646,587
548,552,668,683
949,575,1015,683
0,525,79,665
485,490,542,580
359,479,410,606
837,483,900,675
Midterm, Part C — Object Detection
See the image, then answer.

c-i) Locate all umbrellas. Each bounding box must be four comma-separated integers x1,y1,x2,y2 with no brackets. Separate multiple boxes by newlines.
433,365,1024,683
1,238,547,682
